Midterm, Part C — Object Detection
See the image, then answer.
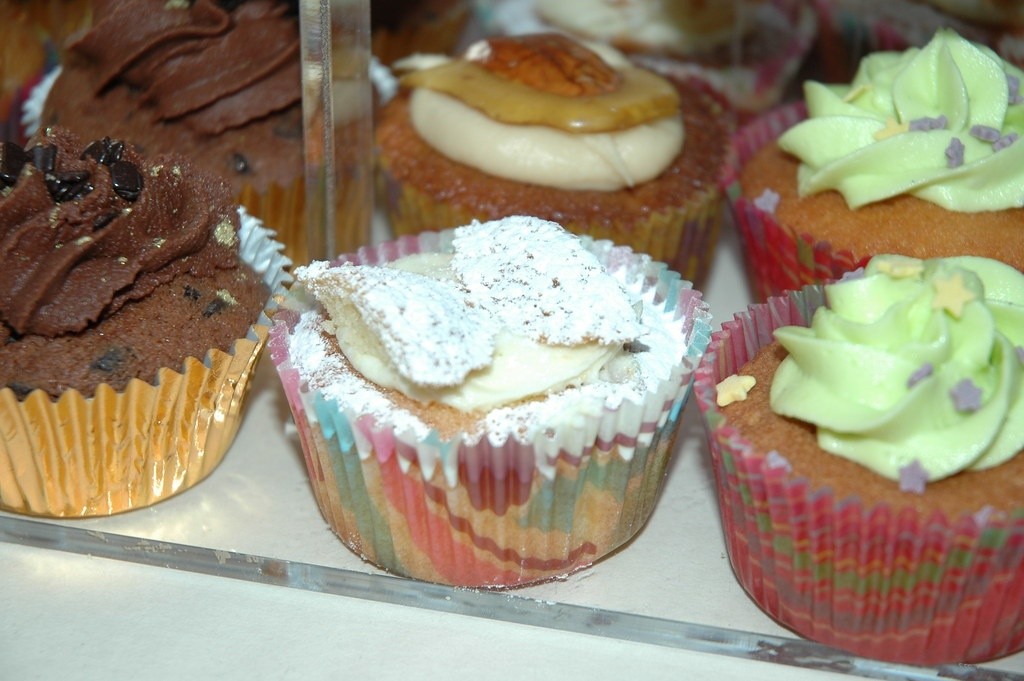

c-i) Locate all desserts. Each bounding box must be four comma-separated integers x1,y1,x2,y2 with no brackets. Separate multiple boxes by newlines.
0,0,1024,670
